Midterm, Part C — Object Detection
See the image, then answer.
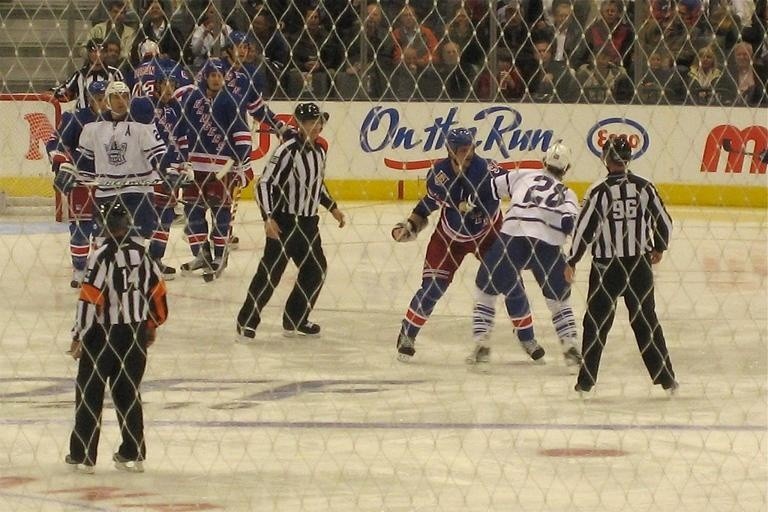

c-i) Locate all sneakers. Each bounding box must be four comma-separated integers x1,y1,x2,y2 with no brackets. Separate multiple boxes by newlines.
65,453,97,467
281,317,320,335
521,340,545,361
70,270,87,288
153,257,176,275
236,320,257,338
574,382,594,392
112,451,147,464
662,379,679,389
203,255,227,279
396,334,416,356
226,234,240,244
180,257,210,270
465,344,490,363
560,343,583,364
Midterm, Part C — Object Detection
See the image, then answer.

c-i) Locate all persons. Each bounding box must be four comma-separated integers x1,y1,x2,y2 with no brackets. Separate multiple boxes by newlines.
565,135,682,402
46,80,113,293
55,37,124,108
180,59,253,282
182,4,233,68
130,0,193,71
391,127,548,364
183,31,302,248
126,67,194,283
78,0,136,71
124,39,193,110
233,101,347,344
63,202,169,473
464,141,584,366
53,82,181,265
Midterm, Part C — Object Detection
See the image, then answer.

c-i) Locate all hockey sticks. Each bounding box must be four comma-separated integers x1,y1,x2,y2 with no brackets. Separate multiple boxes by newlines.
202,181,243,283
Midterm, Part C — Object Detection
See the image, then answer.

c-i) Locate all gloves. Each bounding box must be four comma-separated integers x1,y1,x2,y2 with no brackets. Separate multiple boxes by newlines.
233,166,254,188
162,168,180,192
52,162,79,196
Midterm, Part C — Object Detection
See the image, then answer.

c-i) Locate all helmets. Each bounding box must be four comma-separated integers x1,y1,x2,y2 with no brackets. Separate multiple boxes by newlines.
293,103,328,122
95,201,127,223
446,128,472,149
603,136,632,159
136,40,160,57
226,31,248,48
105,82,131,96
86,36,107,52
204,57,226,74
545,142,569,171
87,38,109,53
87,78,109,95
